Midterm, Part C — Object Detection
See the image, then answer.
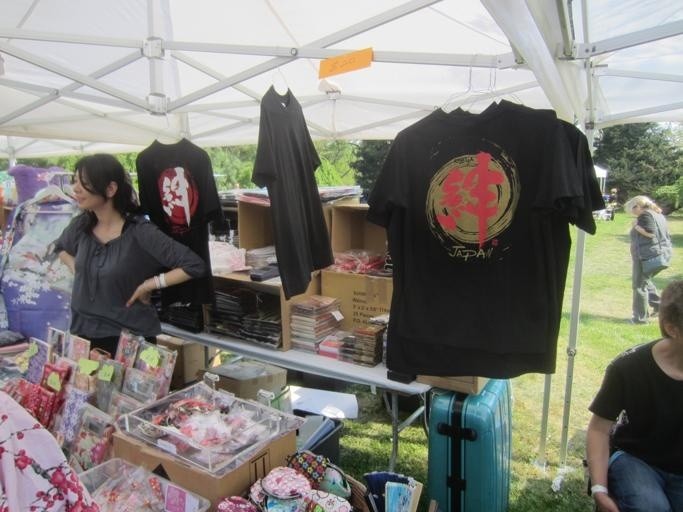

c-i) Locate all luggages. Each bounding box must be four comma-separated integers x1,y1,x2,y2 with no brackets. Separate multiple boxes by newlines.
427,377,512,512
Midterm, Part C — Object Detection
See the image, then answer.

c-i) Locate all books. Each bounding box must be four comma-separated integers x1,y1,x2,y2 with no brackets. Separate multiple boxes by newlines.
2,326,178,473
205,285,391,371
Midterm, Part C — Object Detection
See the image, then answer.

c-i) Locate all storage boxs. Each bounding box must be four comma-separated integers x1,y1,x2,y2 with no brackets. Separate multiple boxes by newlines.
415,374,491,396
197,360,288,400
269,386,293,414
327,194,389,268
110,426,298,507
319,270,393,333
236,189,342,268
292,408,344,467
203,268,320,352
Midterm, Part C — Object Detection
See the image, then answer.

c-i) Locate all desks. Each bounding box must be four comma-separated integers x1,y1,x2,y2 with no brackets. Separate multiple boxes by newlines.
158,323,432,471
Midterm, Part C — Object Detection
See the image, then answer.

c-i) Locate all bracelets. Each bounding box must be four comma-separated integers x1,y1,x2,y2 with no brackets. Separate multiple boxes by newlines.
159,272,166,289
143,280,149,294
152,275,158,290
589,484,608,496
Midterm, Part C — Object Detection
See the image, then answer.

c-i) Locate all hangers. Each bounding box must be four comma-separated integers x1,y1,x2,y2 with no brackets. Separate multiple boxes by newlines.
269,55,293,101
22,174,83,216
431,49,524,114
154,113,187,144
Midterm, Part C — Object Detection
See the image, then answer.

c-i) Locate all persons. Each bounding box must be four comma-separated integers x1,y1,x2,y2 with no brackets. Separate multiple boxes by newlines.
586,281,683,511
608,188,618,220
53,153,206,359
623,193,673,326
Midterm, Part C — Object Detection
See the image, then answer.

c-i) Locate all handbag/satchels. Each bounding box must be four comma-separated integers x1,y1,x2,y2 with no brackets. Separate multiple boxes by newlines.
641,254,668,275
